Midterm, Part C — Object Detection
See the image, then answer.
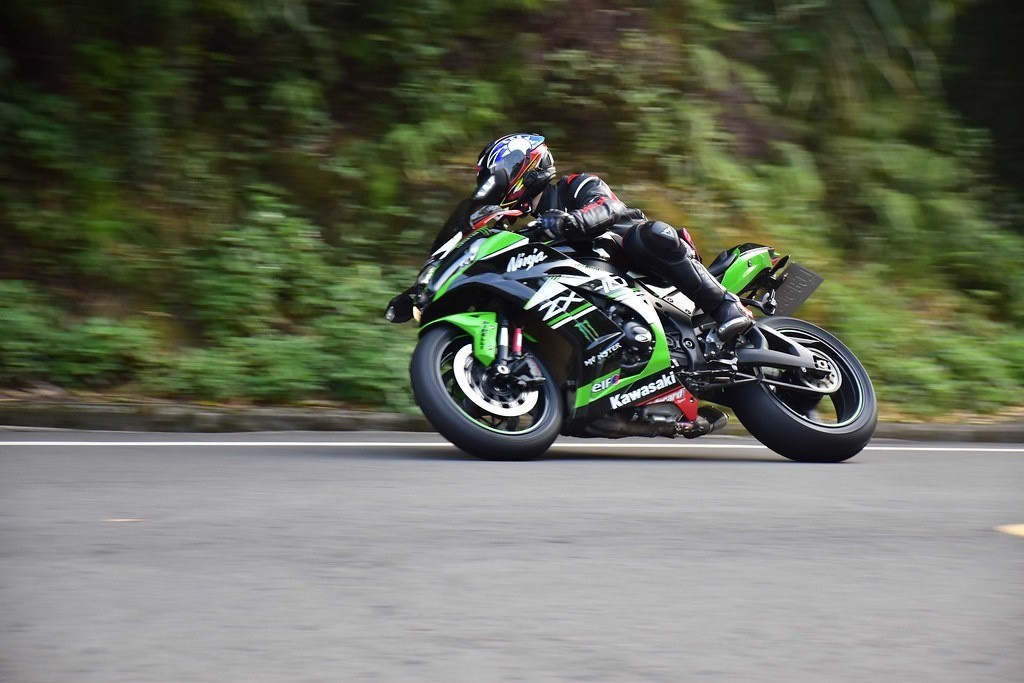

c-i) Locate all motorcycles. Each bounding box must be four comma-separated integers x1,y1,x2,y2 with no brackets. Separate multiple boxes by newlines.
384,169,878,464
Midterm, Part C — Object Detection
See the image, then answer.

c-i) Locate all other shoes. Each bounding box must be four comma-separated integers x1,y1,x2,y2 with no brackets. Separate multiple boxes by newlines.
709,292,756,343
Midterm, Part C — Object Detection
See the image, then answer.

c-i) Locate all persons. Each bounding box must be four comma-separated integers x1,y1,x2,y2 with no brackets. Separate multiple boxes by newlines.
385,133,754,438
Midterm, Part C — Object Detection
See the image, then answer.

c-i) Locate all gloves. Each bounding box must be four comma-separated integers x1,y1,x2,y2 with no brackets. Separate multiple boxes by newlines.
540,208,580,242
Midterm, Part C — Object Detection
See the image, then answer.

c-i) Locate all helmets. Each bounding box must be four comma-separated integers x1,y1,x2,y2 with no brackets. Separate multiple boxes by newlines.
470,133,556,226
